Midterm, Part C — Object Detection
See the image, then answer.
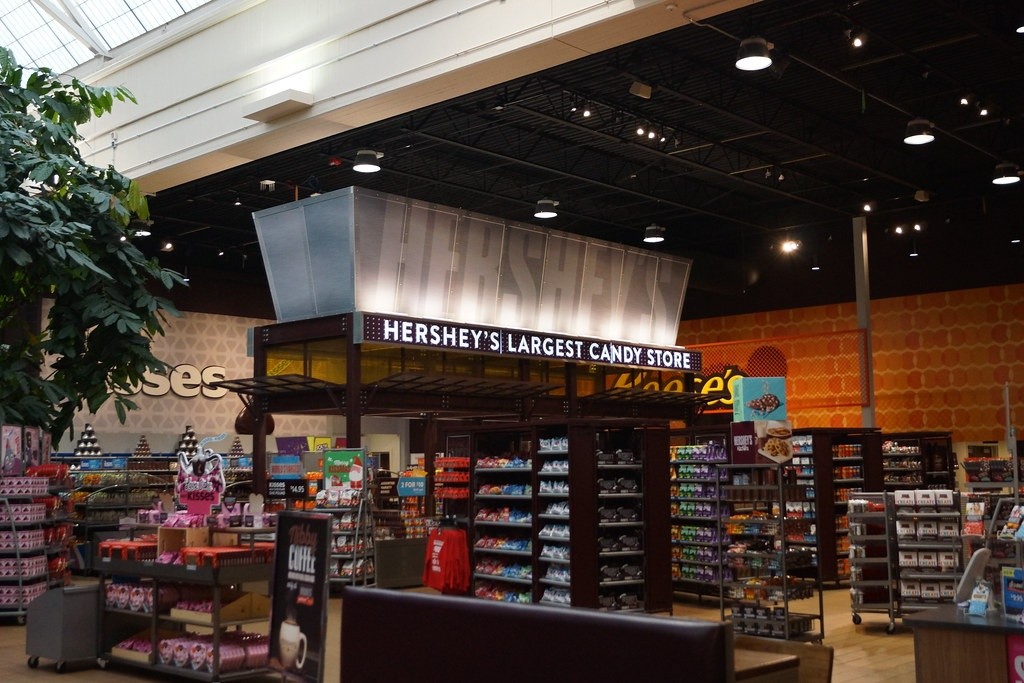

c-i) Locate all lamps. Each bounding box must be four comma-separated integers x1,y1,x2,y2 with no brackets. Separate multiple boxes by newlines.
215,247,225,256
493,93,505,111
909,238,919,257
642,222,667,244
1009,224,1021,245
991,160,1021,185
235,397,275,435
735,36,773,72
234,196,243,206
811,253,820,271
532,199,560,220
624,77,653,101
902,118,935,146
569,91,578,113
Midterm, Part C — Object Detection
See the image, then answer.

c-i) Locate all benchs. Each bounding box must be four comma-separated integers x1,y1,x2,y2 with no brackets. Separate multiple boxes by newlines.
340,584,798,683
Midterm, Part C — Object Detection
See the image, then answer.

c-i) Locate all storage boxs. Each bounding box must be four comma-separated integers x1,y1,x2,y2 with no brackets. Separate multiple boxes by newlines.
170,590,272,625
935,489,954,506
111,626,188,664
901,580,920,597
895,520,915,535
918,552,938,567
894,490,915,507
917,521,938,535
939,552,959,568
898,550,918,566
915,489,936,507
939,582,959,598
921,583,940,598
939,522,960,537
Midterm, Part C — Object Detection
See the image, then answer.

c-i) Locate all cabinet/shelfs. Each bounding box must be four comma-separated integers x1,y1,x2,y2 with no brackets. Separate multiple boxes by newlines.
424,413,964,646
0,482,72,616
50,455,195,577
96,568,276,683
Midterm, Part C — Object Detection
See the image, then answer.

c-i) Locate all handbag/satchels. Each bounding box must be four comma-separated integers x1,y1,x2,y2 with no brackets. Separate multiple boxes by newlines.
422,520,472,594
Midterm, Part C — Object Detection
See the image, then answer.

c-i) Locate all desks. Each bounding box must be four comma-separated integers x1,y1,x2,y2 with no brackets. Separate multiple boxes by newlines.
903,595,1024,683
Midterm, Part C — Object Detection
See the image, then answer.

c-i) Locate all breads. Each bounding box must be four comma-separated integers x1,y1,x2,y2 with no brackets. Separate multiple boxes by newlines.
764,427,791,456
